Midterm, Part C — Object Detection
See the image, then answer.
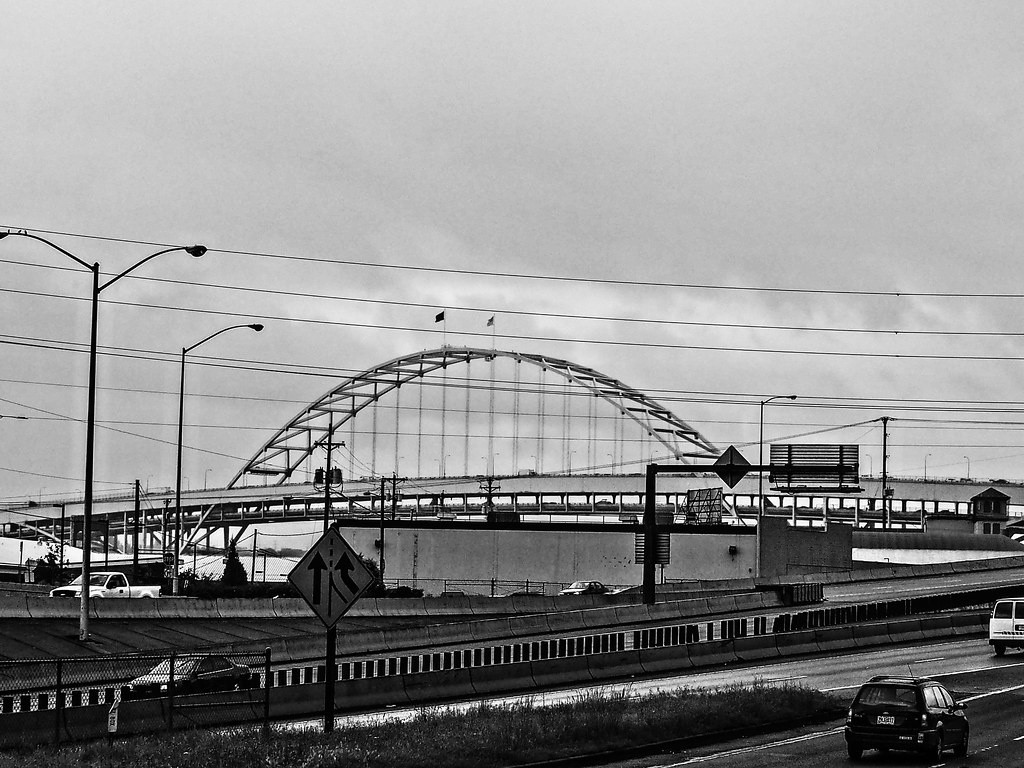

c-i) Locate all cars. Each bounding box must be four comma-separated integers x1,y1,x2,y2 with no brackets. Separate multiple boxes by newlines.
128,653,253,698
558,581,610,596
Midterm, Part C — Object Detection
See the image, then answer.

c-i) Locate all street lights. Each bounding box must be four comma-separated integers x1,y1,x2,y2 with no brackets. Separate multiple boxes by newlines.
866,454,872,476
173,324,264,596
925,453,931,480
53,503,65,587
759,395,798,516
0,231,206,641
964,456,969,480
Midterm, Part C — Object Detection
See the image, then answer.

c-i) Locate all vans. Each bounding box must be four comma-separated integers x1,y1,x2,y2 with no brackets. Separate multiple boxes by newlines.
988,598,1024,655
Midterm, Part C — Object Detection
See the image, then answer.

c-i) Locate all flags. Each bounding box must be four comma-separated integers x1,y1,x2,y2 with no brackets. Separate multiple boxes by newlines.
435,310,445,322
487,317,494,326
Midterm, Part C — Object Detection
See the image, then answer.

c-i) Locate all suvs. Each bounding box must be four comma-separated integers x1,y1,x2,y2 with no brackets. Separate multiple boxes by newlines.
845,674,970,763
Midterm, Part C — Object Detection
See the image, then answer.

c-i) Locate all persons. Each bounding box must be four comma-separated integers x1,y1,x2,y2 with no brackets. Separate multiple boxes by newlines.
110,576,122,588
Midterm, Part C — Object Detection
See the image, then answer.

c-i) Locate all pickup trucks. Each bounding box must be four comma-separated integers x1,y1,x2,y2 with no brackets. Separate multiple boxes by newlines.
49,572,162,599
